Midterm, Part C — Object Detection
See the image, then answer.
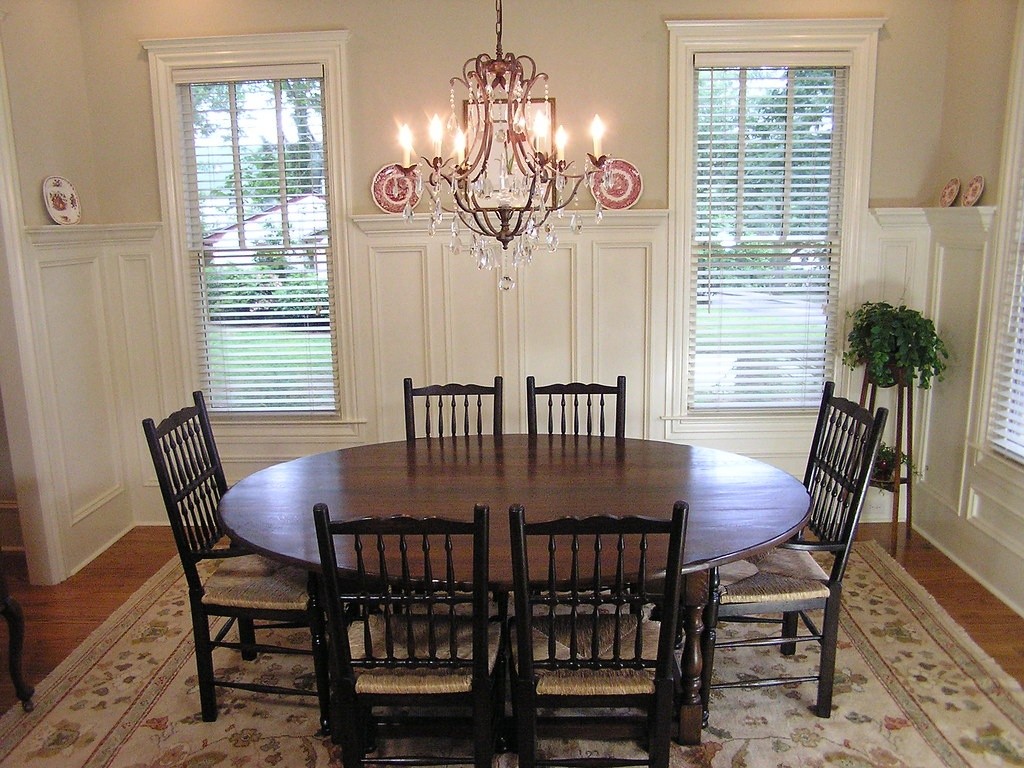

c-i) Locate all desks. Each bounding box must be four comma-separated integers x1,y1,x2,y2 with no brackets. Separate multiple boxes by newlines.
217,434,811,745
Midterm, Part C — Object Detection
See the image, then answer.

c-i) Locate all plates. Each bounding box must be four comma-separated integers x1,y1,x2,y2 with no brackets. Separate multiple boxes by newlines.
938,177,961,208
371,163,423,213
961,175,984,207
590,159,643,209
43,176,82,226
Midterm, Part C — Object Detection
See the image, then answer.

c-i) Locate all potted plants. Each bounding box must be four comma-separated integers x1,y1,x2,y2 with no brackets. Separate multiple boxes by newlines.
869,440,924,497
842,299,948,390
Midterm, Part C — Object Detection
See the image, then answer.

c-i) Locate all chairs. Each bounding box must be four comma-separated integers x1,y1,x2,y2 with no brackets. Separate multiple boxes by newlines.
314,501,507,768
141,390,333,740
508,499,689,768
645,380,889,728
527,376,626,438
392,375,502,615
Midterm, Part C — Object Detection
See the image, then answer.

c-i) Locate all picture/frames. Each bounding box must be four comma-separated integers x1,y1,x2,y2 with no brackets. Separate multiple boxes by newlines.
464,96,556,210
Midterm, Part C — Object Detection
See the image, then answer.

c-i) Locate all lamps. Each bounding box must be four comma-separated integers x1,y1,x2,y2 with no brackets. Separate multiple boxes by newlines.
395,0,608,291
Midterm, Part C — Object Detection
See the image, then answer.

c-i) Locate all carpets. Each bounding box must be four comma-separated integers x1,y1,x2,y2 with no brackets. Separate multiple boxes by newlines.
0,544,1024,768
